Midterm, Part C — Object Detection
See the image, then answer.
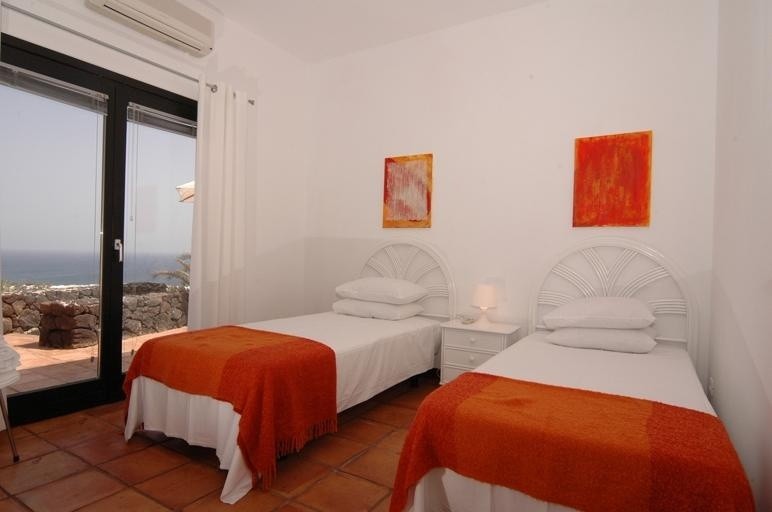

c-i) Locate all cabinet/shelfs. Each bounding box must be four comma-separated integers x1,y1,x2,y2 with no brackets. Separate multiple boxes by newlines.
440,318,522,389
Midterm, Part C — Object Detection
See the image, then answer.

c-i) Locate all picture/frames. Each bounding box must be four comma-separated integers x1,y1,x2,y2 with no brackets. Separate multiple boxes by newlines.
382,152,434,228
571,129,651,226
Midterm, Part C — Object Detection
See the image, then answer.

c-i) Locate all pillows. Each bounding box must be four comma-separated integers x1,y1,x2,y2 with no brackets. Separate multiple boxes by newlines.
542,294,656,328
332,299,424,321
334,277,427,304
549,330,656,354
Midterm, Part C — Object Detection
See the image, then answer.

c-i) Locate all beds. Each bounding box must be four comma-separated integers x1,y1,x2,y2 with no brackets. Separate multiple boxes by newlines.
391,242,759,512
123,238,456,505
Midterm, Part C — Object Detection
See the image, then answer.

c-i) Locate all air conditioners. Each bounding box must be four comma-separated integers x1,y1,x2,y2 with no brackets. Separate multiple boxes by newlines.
69,2,213,59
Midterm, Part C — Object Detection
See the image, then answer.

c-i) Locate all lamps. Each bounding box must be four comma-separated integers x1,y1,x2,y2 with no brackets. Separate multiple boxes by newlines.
469,283,498,326
